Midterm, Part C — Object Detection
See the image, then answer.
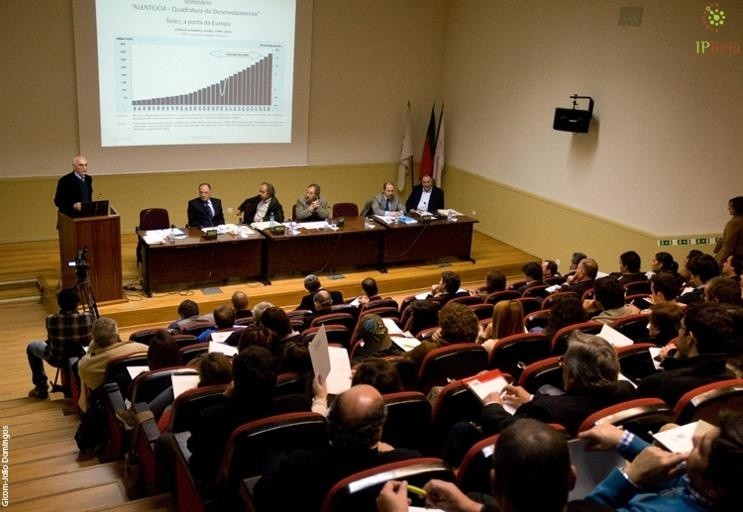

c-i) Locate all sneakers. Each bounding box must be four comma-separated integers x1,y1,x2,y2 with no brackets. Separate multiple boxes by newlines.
29,387,48,399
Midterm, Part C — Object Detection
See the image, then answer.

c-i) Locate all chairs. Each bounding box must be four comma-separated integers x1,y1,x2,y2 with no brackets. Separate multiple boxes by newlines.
139,207,171,230
332,202,359,217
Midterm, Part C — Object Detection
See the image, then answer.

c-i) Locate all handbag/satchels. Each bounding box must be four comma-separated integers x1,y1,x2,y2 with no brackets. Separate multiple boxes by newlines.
50,383,64,392
75,386,108,449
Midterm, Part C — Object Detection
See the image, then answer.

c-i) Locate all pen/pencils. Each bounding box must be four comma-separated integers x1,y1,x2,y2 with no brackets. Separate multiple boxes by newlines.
500,382,514,400
408,485,428,498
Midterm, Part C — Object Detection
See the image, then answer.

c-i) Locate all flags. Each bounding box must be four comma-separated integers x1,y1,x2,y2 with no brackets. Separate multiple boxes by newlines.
396,108,415,192
432,110,445,188
419,108,436,180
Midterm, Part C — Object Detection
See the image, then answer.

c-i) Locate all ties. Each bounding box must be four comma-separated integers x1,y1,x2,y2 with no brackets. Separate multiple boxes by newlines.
81,176,86,185
385,199,389,211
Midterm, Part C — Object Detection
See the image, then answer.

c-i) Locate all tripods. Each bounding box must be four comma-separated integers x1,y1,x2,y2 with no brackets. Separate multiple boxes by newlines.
53,279,101,392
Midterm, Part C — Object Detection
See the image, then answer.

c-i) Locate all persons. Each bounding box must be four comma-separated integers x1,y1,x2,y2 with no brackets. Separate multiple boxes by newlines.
302,290,342,331
406,174,445,213
88,330,183,413
560,257,598,296
445,330,636,463
376,418,593,512
54,156,93,216
478,299,528,358
154,345,312,485
404,300,439,338
703,276,743,315
371,181,406,217
295,183,330,221
519,262,549,296
27,287,94,400
563,253,586,279
678,249,704,277
720,305,743,380
645,303,684,346
350,313,403,367
477,270,507,301
426,270,469,309
239,326,279,355
277,345,314,374
226,302,273,346
579,412,743,512
400,302,479,384
636,303,736,407
580,276,642,327
252,383,422,512
645,252,678,279
187,183,225,227
640,272,687,314
114,352,234,436
260,307,302,353
235,182,284,223
310,357,404,422
232,291,253,320
197,305,236,342
296,274,345,310
169,300,215,334
76,316,150,412
675,255,718,303
610,251,647,286
346,277,392,313
541,260,557,281
530,295,587,335
721,254,743,283
714,195,743,268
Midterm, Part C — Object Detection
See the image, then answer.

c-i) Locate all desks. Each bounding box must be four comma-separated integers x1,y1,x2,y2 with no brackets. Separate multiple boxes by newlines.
250,216,386,284
136,223,268,296
370,208,480,273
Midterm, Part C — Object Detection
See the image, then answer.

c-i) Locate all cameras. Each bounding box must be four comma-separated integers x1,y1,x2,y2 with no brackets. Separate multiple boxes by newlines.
66,247,97,280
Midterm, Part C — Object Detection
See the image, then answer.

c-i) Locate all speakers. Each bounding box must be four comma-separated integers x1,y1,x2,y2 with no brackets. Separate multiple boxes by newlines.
553,107,591,133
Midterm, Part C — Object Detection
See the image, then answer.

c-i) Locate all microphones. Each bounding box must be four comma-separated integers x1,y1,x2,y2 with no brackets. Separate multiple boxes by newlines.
328,205,344,226
209,213,214,230
424,201,428,215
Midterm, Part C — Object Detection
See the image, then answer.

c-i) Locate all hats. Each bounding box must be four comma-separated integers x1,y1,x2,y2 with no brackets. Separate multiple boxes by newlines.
206,202,212,218
359,314,392,352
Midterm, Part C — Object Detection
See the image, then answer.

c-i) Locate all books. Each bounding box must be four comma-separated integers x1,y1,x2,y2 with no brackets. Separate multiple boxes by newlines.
397,216,418,224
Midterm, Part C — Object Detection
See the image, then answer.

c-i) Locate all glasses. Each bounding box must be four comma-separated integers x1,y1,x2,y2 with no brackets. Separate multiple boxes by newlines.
557,357,565,367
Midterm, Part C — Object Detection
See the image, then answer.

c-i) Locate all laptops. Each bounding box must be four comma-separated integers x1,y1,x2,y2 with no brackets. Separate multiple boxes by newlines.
80,200,109,217
